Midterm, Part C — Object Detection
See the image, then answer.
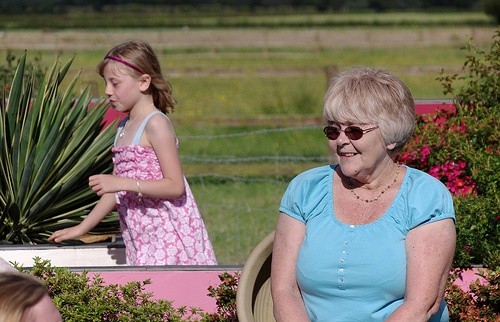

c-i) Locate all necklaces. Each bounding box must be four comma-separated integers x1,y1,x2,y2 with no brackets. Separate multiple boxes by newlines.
346,160,399,201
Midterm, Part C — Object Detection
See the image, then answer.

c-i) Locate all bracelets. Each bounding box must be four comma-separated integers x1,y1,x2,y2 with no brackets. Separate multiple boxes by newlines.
134,178,143,199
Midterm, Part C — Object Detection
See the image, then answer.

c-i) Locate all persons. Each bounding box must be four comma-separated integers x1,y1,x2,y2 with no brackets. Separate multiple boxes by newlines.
0,272,66,322
43,39,218,266
272,66,457,322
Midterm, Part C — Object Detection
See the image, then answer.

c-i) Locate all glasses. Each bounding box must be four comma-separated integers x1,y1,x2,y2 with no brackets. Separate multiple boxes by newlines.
323,125,378,141
